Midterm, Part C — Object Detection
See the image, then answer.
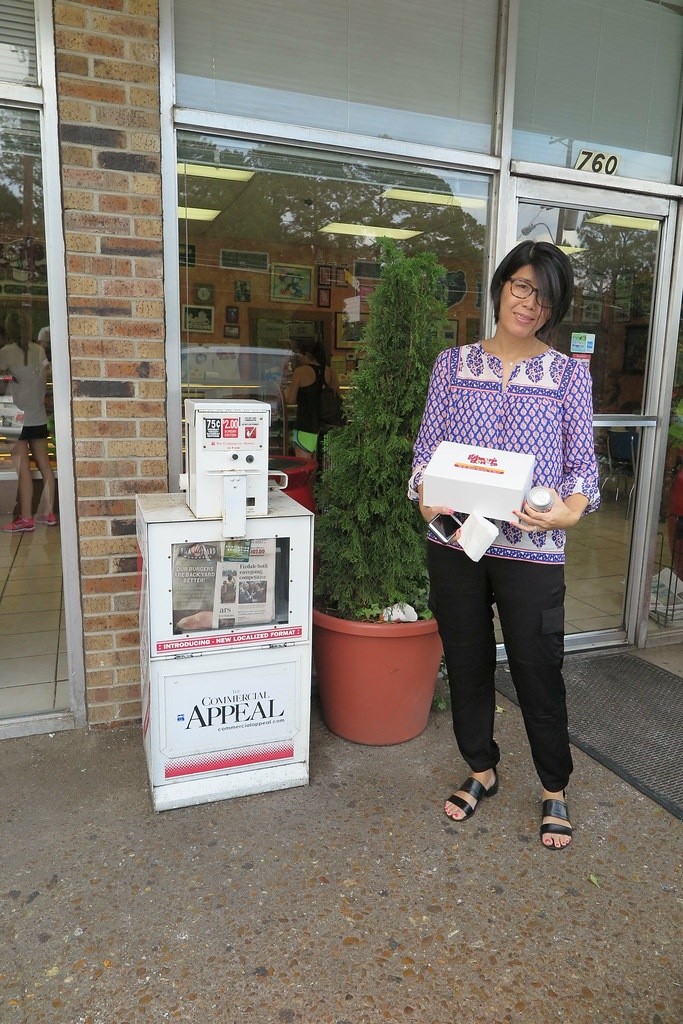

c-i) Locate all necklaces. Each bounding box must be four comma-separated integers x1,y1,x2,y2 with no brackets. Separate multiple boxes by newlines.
510,339,538,365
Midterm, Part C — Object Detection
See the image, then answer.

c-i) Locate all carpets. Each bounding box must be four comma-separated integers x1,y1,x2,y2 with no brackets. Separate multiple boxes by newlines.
495,652,683,822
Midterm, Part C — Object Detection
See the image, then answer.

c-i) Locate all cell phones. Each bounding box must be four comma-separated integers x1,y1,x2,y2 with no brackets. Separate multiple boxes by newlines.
428,514,463,545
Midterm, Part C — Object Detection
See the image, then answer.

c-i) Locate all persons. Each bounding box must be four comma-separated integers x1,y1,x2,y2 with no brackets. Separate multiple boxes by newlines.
187,309,209,323
37,326,49,340
0,309,56,533
221,573,236,602
240,581,266,603
407,240,600,851
277,341,340,458
666,398,683,580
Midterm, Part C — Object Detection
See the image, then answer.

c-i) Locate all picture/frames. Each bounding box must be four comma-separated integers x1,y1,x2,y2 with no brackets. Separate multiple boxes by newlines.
623,324,650,375
612,271,636,323
561,296,575,322
318,266,332,286
336,268,349,287
270,262,314,304
335,312,369,349
318,288,331,308
436,319,459,347
179,243,197,269
182,304,215,333
580,274,605,324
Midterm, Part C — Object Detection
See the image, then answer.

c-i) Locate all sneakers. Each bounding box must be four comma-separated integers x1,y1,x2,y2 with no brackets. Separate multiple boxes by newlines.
35,513,57,525
1,512,35,532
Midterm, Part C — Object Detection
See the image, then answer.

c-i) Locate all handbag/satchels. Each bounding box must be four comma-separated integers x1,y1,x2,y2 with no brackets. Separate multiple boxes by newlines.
322,387,344,428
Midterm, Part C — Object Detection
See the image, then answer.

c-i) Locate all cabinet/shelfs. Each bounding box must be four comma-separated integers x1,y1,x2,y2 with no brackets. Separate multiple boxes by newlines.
183,380,360,456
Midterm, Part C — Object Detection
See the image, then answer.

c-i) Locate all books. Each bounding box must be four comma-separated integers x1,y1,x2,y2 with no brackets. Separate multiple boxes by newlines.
650,568,683,626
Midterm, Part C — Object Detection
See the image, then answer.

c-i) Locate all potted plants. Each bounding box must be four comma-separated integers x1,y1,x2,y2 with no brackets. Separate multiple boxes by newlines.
313,237,443,747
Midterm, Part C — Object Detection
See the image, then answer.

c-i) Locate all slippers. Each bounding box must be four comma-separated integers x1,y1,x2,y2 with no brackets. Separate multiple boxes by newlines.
540,790,572,850
445,766,498,821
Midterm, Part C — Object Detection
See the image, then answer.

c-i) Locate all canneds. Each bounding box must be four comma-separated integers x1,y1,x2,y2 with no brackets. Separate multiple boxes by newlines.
524,486,552,516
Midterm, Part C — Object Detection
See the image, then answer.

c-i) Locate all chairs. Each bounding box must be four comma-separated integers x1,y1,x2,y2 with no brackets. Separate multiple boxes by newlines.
601,429,639,520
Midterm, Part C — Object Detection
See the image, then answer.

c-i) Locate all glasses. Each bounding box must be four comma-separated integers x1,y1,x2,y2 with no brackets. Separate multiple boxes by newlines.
506,276,555,309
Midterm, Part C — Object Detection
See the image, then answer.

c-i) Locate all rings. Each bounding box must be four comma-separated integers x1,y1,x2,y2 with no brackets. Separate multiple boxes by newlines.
535,526,537,532
